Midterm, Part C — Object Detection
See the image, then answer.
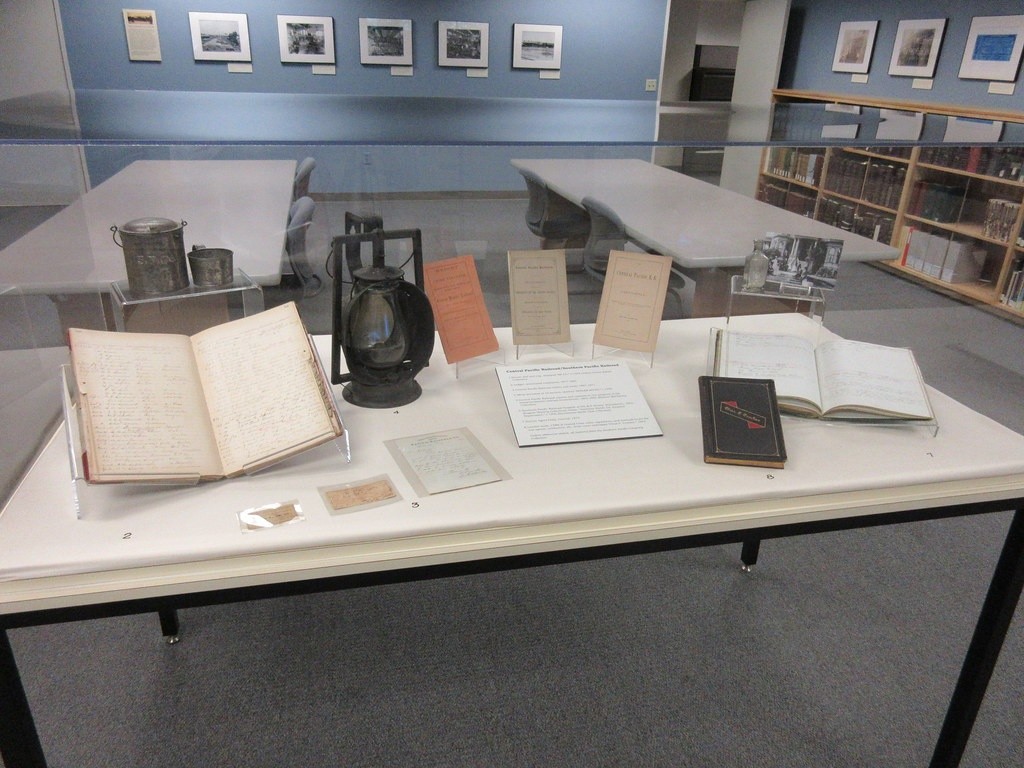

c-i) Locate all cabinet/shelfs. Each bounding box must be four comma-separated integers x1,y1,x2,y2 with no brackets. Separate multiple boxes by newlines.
757,90,1024,326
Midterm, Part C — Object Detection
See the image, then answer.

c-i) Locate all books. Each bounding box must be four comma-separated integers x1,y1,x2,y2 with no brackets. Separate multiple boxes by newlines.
698,325,938,471
757,145,1024,312
69,301,343,484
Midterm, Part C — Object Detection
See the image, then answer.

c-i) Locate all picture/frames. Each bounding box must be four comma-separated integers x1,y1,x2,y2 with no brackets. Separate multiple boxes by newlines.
277,15,335,63
887,19,946,78
957,14,1024,83
189,12,251,61
359,18,412,64
437,20,489,68
832,20,879,72
512,23,562,70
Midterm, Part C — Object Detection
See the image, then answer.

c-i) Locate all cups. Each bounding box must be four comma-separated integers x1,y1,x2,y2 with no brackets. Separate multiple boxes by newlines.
189,243,234,285
113,217,190,293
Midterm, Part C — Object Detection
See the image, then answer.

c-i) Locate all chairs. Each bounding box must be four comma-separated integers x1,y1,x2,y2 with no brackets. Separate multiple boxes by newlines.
581,196,685,321
283,157,324,298
517,164,591,272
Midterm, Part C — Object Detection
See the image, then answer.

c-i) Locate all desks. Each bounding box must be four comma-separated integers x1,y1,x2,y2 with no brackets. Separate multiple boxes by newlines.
510,158,901,320
0,160,298,350
0,314,1024,768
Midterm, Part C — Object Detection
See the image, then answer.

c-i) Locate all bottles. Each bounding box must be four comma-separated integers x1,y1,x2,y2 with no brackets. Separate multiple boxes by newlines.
744,240,768,287
